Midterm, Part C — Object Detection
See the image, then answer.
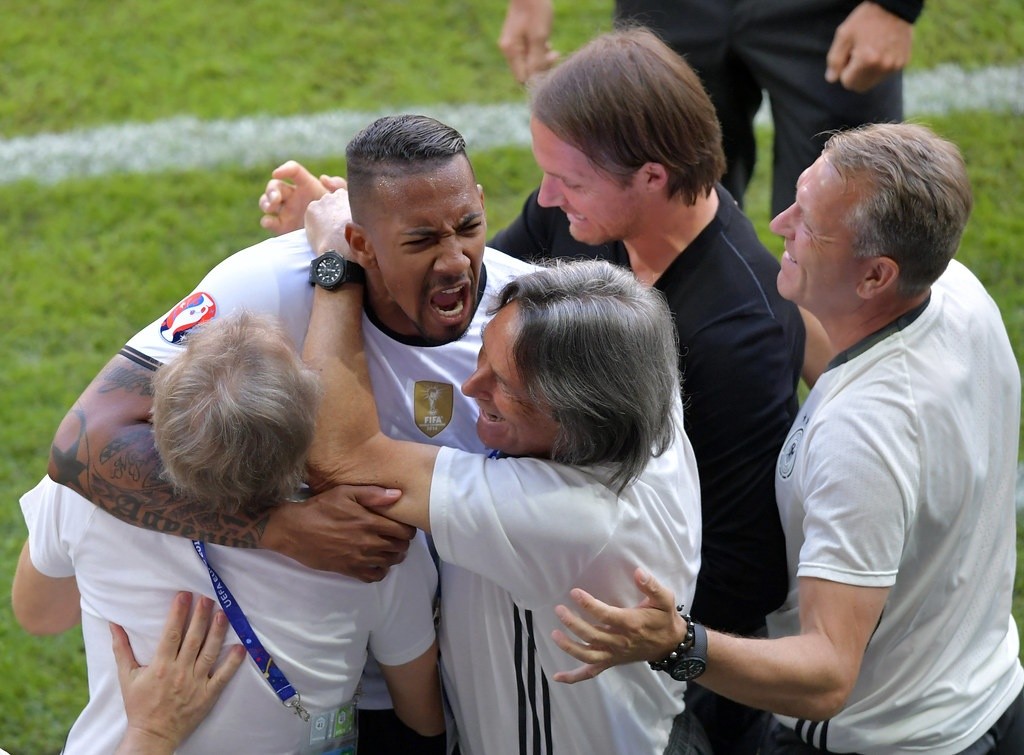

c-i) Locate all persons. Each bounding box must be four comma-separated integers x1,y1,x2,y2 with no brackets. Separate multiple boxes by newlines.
260,160,703,755
13,321,446,755
109,591,248,755
46,113,550,583
499,0,927,238
552,123,1024,755
486,32,805,755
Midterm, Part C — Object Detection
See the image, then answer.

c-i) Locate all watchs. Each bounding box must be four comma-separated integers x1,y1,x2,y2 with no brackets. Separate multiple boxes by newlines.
309,250,368,290
664,624,708,681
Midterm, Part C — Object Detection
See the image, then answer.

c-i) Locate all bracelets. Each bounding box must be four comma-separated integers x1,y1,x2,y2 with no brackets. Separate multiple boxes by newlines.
646,605,695,671
